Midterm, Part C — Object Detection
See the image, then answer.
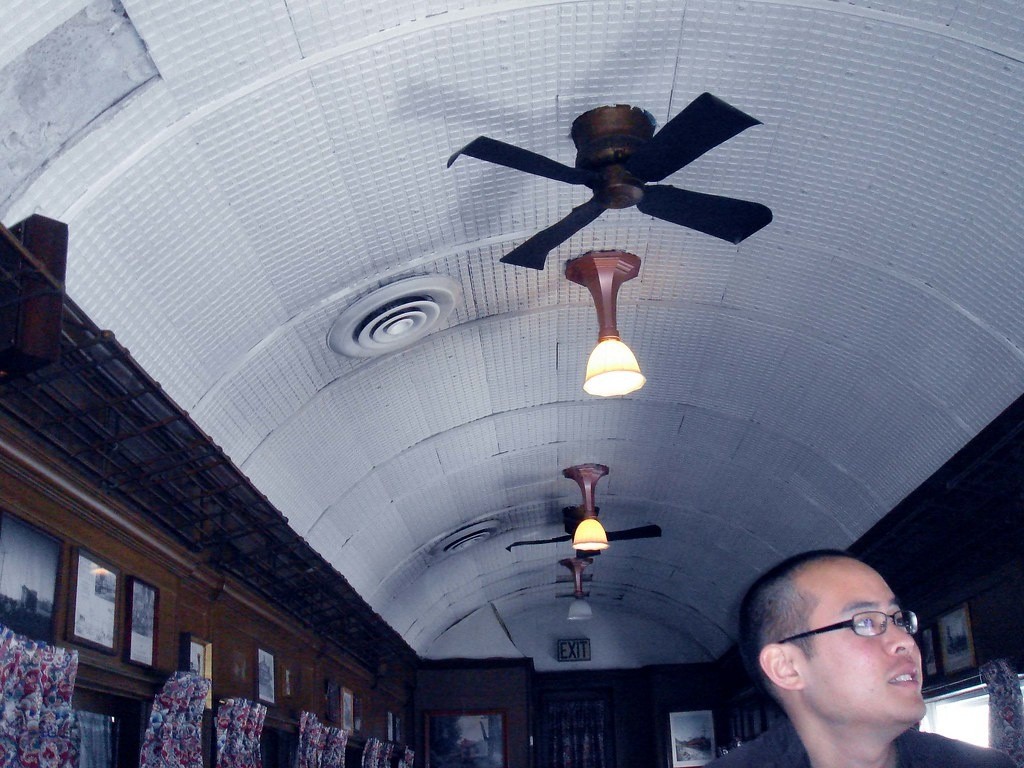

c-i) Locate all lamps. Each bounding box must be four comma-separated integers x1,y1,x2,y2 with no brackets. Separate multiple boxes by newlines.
563,250,647,397
558,558,596,623
561,463,611,552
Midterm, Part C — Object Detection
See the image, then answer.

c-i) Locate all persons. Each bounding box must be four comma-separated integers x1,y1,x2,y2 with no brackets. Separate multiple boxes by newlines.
696,547,1015,768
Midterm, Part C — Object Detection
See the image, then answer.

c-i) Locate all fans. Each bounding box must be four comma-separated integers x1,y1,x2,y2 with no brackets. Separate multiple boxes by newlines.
505,505,662,551
447,92,775,270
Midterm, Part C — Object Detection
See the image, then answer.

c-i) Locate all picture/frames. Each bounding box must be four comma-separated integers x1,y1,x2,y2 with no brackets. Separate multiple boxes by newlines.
383,712,396,743
253,646,279,708
66,544,122,656
394,715,402,745
181,632,214,712
932,601,978,677
663,708,720,768
122,574,161,671
339,686,355,735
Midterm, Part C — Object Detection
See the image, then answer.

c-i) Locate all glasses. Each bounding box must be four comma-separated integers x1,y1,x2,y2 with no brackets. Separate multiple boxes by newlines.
779,610,918,644
